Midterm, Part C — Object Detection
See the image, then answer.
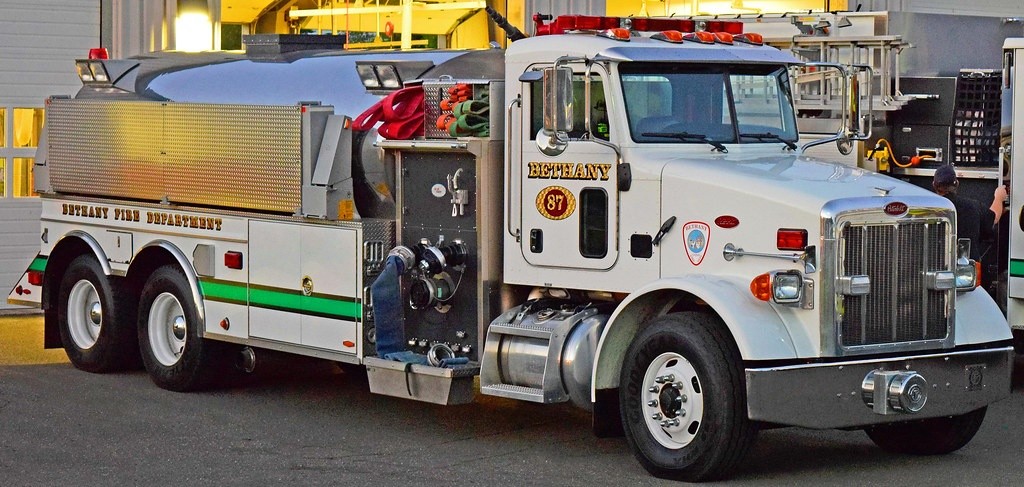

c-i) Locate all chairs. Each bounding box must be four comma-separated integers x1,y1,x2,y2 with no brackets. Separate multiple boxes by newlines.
636,116,680,134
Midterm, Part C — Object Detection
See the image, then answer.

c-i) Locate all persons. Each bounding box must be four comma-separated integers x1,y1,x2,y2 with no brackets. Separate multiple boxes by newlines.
933,166,1007,277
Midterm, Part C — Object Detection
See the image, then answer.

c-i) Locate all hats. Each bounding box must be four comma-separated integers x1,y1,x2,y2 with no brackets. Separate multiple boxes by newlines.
933,165,956,186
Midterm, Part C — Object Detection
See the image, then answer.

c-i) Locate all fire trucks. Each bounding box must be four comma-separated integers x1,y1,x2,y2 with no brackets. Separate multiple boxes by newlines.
723,0,1024,342
8,13,1013,482
